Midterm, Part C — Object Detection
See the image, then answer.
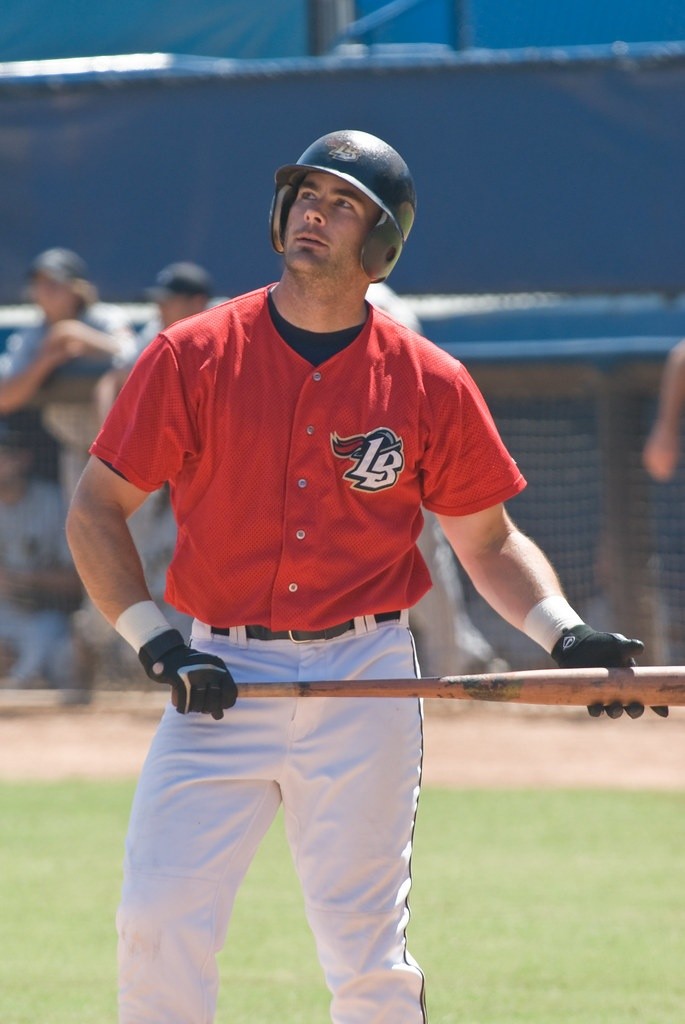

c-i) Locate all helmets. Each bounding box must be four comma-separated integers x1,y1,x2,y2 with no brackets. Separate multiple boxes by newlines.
270,129,416,283
33,245,103,306
146,263,215,296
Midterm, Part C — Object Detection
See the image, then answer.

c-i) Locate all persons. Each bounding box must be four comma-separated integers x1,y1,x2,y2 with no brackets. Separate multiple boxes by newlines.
64,128,669,1024
0,249,684,712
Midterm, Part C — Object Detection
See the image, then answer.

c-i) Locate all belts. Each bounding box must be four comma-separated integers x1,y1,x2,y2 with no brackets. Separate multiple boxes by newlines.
210,609,401,641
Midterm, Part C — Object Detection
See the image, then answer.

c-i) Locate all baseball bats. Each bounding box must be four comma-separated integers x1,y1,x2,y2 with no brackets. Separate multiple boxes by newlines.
171,665,685,706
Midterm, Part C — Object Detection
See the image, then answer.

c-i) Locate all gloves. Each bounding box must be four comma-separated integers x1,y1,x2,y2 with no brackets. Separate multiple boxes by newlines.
143,635,236,719
549,625,671,718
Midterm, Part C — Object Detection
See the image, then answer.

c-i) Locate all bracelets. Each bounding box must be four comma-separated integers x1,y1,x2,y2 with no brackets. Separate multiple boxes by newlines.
113,599,171,653
524,595,586,655
